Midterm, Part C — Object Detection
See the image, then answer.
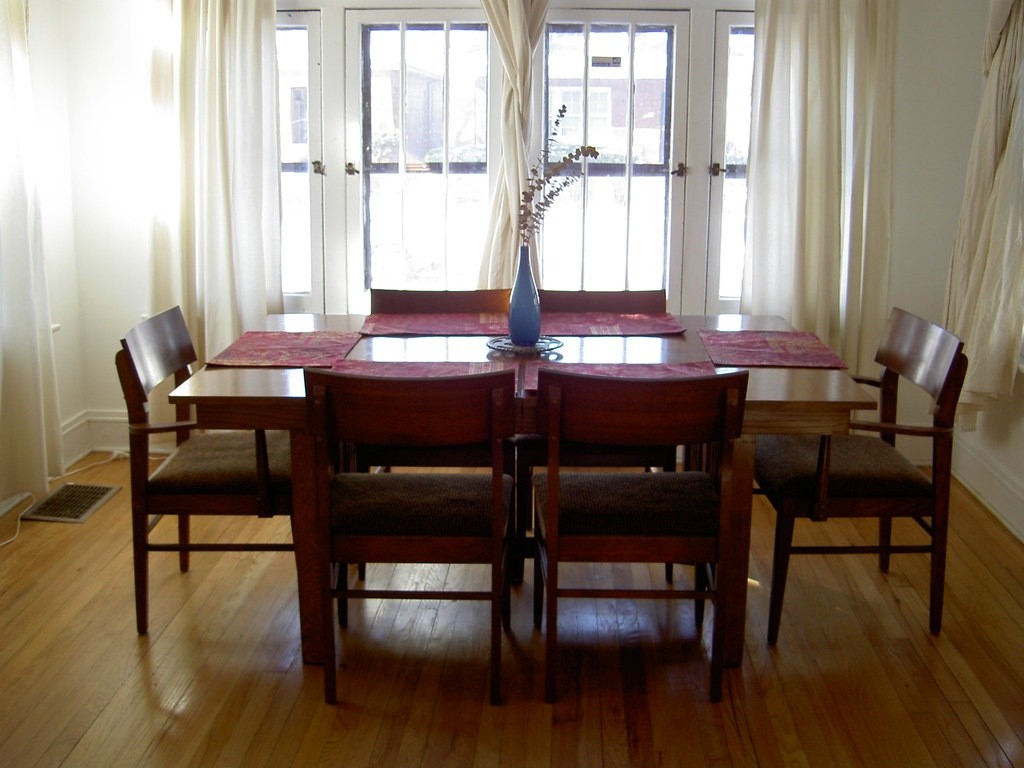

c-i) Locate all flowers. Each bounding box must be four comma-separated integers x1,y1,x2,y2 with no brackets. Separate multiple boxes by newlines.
517,104,599,247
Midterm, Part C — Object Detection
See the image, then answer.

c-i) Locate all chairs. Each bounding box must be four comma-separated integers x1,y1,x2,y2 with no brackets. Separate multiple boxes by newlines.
111,276,971,706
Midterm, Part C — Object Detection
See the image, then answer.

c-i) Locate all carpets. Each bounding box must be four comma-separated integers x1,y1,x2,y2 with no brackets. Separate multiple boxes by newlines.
16,482,123,525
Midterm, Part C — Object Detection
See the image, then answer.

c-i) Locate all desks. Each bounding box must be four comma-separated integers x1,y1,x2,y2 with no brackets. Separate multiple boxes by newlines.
167,311,877,673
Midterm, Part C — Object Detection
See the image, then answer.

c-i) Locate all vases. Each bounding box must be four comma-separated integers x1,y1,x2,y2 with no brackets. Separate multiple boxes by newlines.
505,246,546,346
